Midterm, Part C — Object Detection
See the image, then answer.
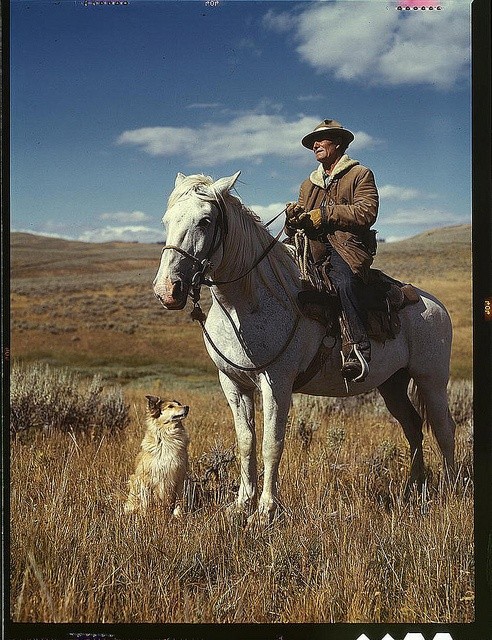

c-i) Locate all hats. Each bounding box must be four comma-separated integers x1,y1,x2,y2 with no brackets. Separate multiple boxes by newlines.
301,118,354,150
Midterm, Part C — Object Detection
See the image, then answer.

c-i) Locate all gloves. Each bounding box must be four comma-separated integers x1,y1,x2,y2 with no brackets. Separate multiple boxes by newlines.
304,208,321,230
286,201,305,228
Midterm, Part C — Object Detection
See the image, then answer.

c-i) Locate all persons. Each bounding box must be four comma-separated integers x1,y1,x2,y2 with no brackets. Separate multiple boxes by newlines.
283,120,380,380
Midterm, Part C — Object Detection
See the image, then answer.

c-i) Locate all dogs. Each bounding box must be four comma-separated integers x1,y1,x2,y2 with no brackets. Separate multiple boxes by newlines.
124,395,190,514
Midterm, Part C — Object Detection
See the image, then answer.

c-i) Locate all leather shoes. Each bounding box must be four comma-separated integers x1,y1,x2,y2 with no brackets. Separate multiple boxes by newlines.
342,358,368,383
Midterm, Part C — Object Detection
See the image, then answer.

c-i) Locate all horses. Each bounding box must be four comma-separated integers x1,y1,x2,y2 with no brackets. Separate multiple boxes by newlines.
153,171,455,525
